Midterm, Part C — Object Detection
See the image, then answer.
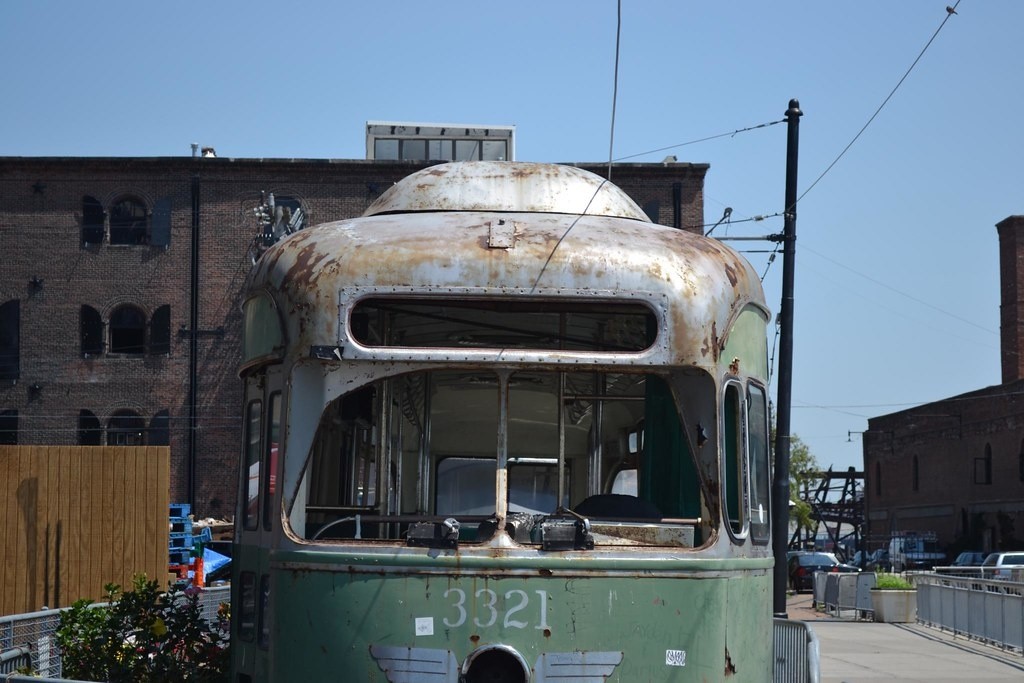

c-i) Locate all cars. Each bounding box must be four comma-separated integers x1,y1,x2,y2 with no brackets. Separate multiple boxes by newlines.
949,551,987,577
980,552,1024,581
786,549,889,593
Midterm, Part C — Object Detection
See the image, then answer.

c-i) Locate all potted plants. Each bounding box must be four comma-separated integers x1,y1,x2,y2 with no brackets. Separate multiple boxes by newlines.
869,565,918,623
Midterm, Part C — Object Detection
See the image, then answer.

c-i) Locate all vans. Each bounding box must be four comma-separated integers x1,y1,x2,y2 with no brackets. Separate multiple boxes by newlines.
888,535,947,574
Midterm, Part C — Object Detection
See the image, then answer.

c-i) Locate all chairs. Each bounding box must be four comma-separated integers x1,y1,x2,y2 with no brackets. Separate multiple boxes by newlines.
576,494,655,517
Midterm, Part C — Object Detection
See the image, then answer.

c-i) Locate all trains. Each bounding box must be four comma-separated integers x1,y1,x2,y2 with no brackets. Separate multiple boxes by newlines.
227,159,776,683
